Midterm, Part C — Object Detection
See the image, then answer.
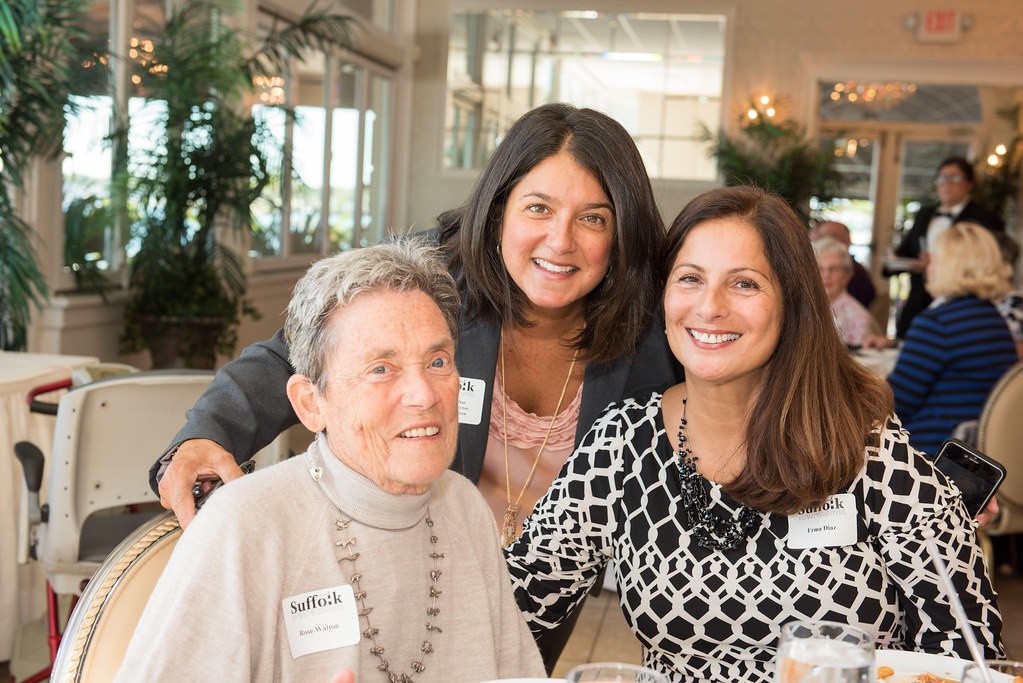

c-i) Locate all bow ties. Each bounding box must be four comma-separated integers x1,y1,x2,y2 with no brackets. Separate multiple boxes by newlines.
934,211,952,217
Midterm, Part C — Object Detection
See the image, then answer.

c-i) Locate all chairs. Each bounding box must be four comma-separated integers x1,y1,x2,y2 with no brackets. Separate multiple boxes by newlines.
13,364,295,597
49,509,183,683
976,360,1023,581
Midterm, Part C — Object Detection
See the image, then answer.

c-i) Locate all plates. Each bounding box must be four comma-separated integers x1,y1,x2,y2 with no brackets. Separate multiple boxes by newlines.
858,347,898,357
881,257,918,270
873,650,1014,683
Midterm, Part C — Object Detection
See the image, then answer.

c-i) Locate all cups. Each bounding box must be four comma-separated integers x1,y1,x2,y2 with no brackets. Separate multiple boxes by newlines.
963,660,1023,683
568,662,671,683
775,620,877,683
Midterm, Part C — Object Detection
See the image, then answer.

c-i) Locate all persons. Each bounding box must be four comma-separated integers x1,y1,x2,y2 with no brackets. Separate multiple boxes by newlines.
863,156,1023,351
503,186,1010,682
148,105,999,675
112,238,549,683
886,223,1020,461
806,221,881,352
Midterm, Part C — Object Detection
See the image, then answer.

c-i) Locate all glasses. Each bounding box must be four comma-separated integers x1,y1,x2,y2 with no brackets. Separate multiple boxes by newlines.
934,175,964,185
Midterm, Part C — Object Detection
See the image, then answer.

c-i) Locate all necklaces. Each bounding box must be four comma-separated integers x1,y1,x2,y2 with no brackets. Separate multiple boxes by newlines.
502,322,587,543
336,505,445,683
676,398,764,550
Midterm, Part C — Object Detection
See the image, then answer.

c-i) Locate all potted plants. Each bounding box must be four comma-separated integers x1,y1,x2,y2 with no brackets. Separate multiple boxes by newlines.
63,0,374,370
